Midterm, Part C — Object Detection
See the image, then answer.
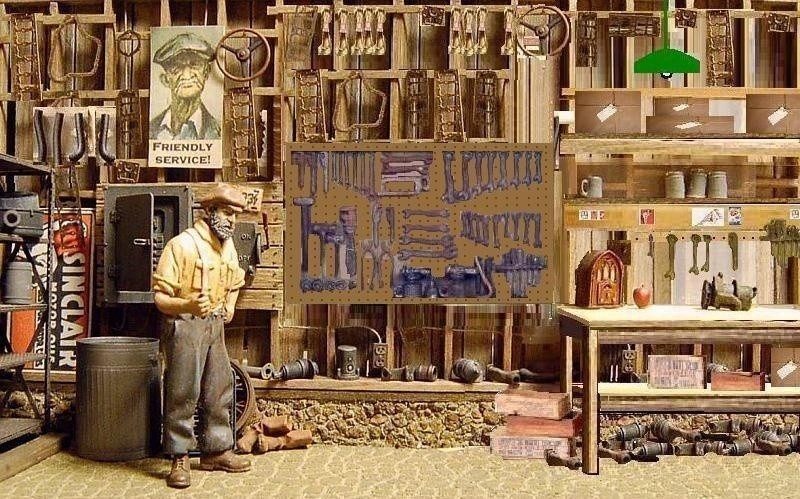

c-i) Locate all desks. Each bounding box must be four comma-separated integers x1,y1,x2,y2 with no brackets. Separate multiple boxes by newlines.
557,305,800,475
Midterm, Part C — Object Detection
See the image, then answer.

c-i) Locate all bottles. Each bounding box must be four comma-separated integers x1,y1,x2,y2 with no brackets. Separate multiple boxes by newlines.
662,170,728,199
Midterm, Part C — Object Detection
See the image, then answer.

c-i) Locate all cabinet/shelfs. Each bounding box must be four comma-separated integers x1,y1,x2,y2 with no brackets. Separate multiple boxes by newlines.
560,87,800,242
0,152,57,446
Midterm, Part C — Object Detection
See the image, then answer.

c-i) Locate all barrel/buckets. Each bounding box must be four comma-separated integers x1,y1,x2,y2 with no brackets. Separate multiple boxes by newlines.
76,336,161,461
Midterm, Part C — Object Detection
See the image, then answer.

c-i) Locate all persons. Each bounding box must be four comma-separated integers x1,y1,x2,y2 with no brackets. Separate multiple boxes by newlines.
153,183,258,489
148,30,221,141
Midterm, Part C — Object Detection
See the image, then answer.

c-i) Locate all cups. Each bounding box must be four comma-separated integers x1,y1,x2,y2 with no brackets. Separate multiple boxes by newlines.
580,176,602,199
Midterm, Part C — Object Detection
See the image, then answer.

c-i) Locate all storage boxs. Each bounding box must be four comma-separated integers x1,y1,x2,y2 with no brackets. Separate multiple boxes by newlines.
771,348,800,387
711,368,765,391
489,388,582,459
646,354,707,389
575,92,799,134
